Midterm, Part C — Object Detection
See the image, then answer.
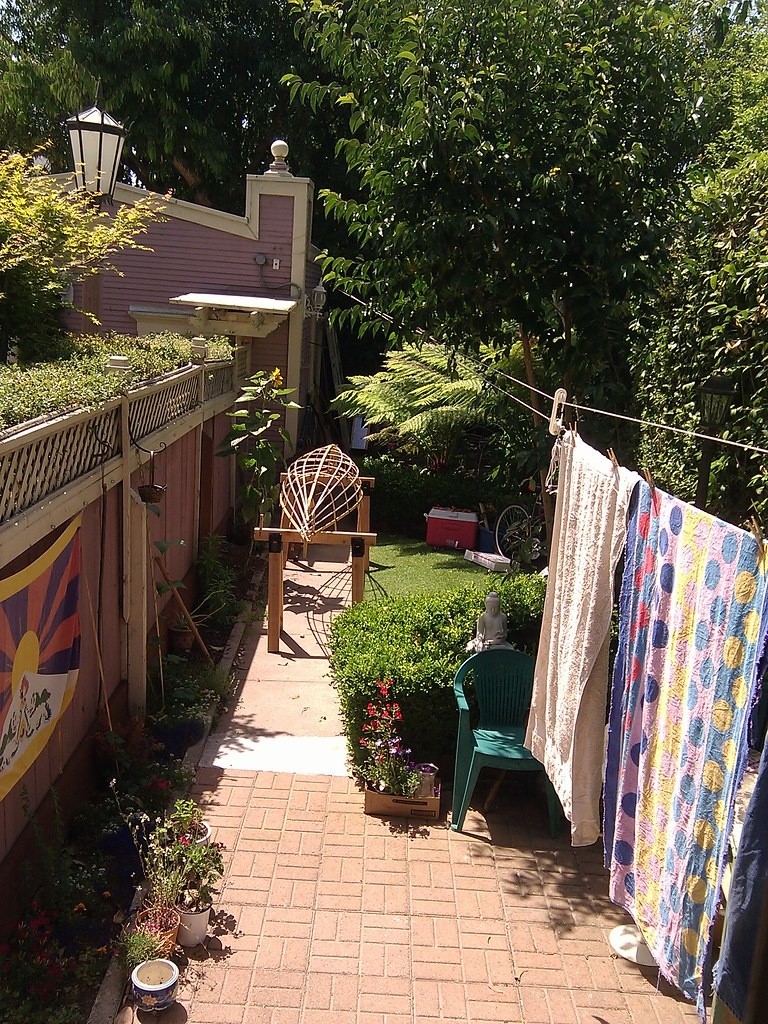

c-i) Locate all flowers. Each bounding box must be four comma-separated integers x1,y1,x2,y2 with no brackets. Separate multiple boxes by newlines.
348,678,440,798
107,777,227,981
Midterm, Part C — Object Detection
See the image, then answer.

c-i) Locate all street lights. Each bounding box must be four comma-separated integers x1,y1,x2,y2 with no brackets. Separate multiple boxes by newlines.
63,78,139,354
696,370,737,510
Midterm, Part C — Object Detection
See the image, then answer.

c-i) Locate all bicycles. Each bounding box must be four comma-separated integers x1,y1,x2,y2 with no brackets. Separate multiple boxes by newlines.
494,468,558,560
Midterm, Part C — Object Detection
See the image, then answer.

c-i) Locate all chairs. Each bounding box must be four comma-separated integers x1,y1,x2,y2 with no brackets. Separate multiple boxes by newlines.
452,647,562,842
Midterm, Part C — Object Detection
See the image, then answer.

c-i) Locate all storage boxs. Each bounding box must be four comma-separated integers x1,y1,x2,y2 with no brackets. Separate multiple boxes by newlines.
476,524,515,558
364,777,442,821
425,505,479,552
464,549,520,573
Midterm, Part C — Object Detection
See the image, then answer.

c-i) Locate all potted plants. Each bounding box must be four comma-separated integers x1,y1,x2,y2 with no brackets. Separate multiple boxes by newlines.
158,589,226,649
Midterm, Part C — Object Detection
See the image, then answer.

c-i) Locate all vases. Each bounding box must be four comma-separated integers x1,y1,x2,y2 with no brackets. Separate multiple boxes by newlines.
132,957,179,1011
135,907,181,952
174,889,213,947
177,821,212,846
